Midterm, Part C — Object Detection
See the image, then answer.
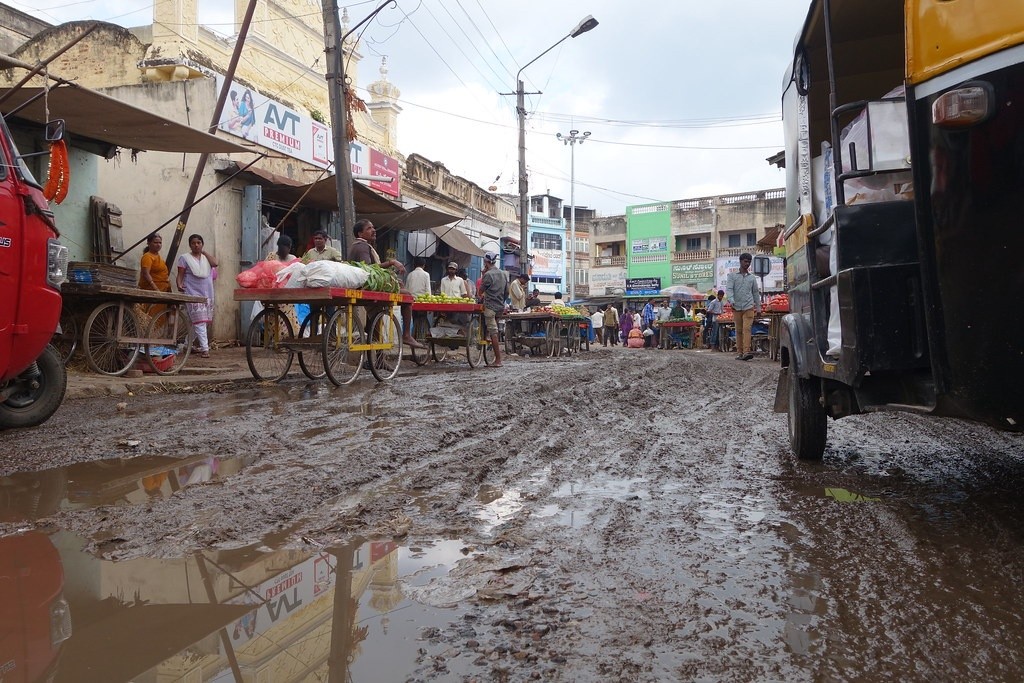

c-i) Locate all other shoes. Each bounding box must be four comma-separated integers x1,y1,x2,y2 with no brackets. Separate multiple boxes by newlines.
735,354,743,360
742,353,753,360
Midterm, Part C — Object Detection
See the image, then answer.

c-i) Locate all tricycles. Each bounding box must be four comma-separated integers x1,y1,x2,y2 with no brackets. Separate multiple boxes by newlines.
0,107,75,422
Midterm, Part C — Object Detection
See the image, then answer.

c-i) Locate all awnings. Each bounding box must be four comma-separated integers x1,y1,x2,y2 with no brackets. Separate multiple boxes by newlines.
427,225,491,257
0,80,267,266
264,170,405,212
385,205,463,266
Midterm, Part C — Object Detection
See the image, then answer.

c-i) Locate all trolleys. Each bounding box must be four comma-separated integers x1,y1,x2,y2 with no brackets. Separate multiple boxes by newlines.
657,309,784,363
61,281,209,379
233,290,419,387
421,304,501,369
506,313,592,359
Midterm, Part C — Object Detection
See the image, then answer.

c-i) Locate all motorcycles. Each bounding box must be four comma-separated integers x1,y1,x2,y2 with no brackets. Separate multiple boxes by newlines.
768,0,1024,461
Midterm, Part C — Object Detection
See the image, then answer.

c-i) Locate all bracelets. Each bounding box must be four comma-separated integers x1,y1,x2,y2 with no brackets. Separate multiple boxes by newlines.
389,259,391,266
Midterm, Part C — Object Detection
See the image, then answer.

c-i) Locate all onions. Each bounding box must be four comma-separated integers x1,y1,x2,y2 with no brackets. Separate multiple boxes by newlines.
761,293,788,312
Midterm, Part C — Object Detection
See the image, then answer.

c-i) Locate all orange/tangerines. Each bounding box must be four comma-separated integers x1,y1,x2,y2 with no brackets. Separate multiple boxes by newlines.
553,305,578,315
415,291,474,303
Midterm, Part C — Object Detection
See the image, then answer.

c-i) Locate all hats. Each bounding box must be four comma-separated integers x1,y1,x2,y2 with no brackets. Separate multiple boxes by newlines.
448,262,458,270
484,252,499,262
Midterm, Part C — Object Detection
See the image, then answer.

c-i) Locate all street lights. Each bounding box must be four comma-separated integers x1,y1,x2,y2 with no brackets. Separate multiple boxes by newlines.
553,129,593,306
513,14,600,289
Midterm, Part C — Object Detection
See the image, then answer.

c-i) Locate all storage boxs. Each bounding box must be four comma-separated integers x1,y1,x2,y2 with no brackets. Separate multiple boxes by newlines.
120,353,175,372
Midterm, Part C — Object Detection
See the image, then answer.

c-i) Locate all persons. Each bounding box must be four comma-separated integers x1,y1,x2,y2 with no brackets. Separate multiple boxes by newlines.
300,231,340,341
708,290,725,351
139,234,170,343
702,294,716,349
585,299,703,351
440,261,477,297
727,253,760,361
524,289,540,308
177,234,218,358
477,251,509,367
509,275,531,311
264,233,300,341
349,219,424,371
643,296,656,348
228,89,254,130
825,136,905,355
405,256,431,297
551,291,565,307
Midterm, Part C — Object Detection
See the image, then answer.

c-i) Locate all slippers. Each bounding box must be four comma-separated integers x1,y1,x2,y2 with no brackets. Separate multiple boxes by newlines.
201,353,209,358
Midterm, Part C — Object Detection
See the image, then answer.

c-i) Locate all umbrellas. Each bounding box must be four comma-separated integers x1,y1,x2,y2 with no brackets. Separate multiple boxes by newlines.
659,285,704,302
